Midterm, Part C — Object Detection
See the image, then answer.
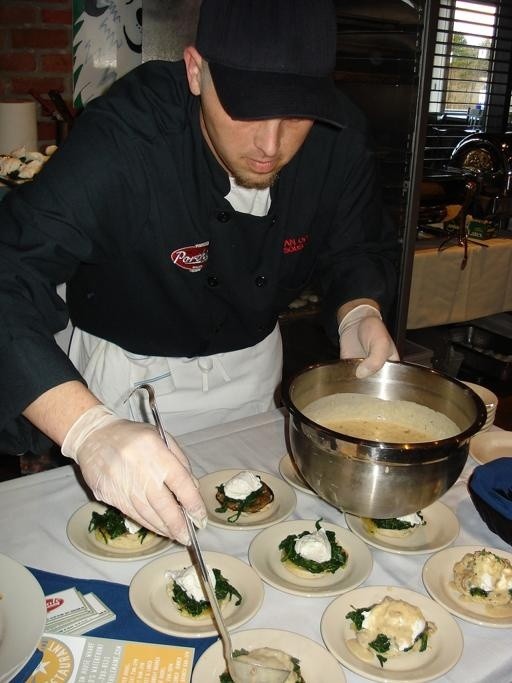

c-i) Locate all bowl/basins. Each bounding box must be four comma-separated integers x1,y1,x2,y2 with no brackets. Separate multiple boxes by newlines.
277,356,488,522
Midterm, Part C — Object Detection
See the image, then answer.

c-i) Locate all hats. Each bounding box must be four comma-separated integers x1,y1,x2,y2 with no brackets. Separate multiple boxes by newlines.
195,0,348,130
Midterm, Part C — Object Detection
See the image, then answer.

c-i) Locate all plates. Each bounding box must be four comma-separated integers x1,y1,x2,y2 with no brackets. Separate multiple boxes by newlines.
192,468,299,531
459,380,498,437
421,543,512,626
190,628,346,682
320,583,464,681
126,546,270,640
244,518,376,601
343,498,463,556
276,451,315,499
64,499,178,563
0,553,48,683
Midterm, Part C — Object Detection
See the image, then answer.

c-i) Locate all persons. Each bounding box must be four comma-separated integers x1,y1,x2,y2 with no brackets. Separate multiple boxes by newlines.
0,0,400,546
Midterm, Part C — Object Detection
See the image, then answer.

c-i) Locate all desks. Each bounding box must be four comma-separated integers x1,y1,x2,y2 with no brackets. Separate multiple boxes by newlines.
0,407,512,683
